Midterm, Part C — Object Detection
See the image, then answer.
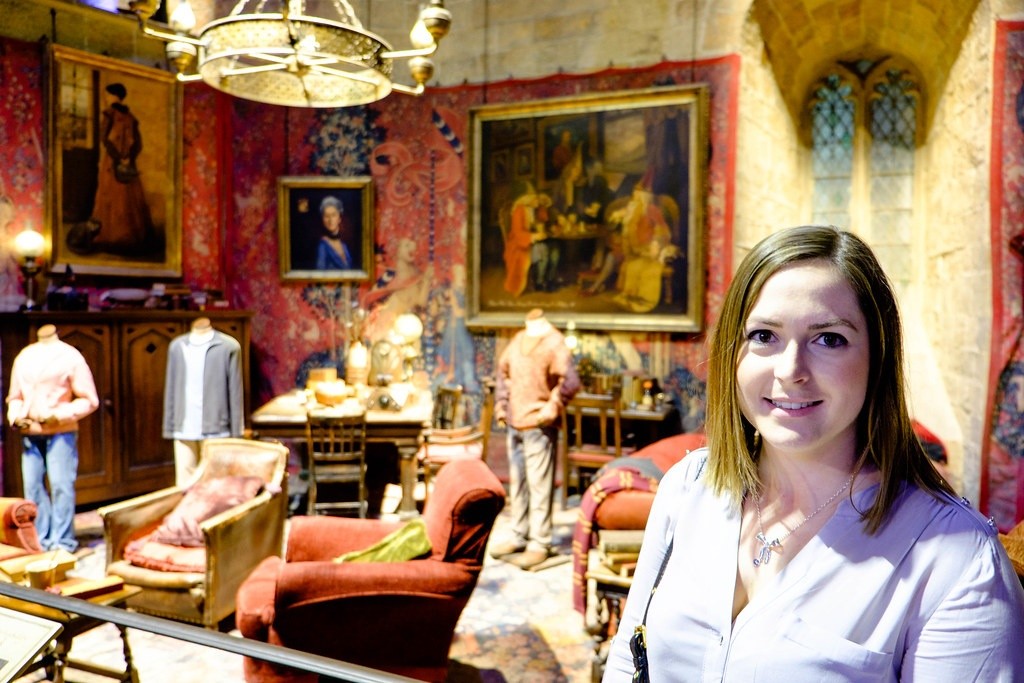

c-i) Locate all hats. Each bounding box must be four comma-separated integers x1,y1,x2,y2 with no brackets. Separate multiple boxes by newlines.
105,82,127,101
320,196,343,216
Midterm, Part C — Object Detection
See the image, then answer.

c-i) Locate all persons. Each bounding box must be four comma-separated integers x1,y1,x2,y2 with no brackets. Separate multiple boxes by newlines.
160,319,243,483
496,309,579,568
599,224,1023,683
4,324,100,553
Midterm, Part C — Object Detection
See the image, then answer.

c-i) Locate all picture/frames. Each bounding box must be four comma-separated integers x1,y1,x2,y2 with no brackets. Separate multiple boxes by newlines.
275,176,376,283
42,33,184,278
464,81,712,332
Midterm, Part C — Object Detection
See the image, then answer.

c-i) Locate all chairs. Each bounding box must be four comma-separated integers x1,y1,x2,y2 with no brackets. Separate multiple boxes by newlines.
558,398,637,510
306,409,368,522
417,385,464,475
412,377,494,511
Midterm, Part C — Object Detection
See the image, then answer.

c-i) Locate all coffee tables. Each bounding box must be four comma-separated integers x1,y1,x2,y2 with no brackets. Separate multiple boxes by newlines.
0,549,144,683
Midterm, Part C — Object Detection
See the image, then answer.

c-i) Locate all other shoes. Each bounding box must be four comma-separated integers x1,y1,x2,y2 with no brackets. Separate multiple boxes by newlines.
520,547,548,568
488,540,516,559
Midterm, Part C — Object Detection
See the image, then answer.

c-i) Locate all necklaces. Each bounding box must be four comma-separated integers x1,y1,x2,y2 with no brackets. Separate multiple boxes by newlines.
753,470,854,567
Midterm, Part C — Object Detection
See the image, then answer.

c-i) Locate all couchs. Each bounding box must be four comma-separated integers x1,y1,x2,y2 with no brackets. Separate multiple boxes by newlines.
569,428,710,637
97,437,290,633
235,454,506,683
0,495,44,580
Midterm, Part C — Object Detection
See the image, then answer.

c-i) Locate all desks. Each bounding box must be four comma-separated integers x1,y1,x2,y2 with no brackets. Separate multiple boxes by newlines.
552,402,671,512
249,370,434,520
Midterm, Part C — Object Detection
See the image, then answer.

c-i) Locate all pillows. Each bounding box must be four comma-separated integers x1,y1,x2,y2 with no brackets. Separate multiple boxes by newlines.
334,516,433,564
152,458,265,546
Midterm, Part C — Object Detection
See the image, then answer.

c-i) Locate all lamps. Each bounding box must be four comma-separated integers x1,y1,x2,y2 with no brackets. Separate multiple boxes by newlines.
12,229,47,310
130,0,452,107
349,342,369,386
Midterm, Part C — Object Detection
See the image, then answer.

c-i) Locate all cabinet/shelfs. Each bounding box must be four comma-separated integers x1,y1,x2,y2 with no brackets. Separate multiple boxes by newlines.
0,308,256,506
